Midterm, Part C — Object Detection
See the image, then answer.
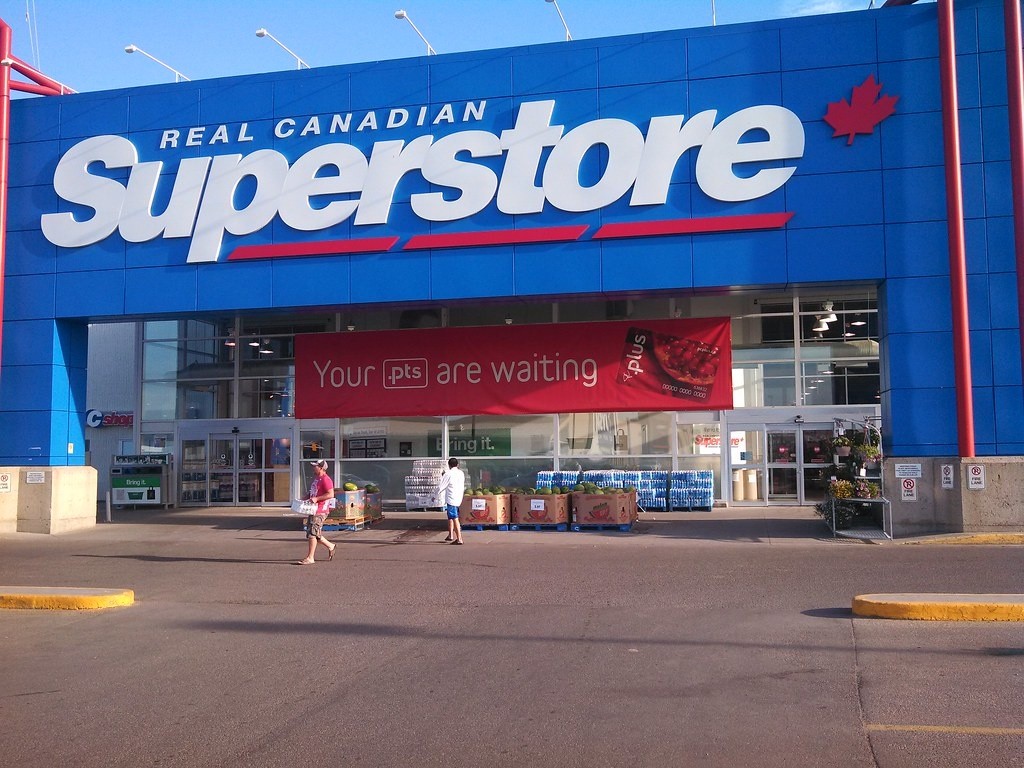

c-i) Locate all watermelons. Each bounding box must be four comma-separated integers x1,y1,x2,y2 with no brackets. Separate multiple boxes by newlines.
359,484,379,493
464,485,636,495
343,482,357,491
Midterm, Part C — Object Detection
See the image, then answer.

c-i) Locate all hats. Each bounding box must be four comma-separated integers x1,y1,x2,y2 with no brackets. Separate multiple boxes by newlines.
310,460,328,470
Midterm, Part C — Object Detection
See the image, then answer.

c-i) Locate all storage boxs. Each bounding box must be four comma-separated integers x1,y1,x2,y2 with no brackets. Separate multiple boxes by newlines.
460,494,511,524
365,492,383,520
329,489,367,519
512,492,569,524
572,491,638,523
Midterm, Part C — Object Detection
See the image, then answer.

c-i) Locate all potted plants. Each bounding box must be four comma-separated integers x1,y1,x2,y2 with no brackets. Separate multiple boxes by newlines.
812,426,881,530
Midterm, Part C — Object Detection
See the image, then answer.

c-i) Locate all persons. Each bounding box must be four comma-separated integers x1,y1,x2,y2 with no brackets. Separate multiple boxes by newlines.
431,457,467,545
296,459,339,565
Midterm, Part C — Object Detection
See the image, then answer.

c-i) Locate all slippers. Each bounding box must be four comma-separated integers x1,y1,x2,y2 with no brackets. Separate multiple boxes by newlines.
329,544,337,561
298,556,315,564
450,540,464,545
445,536,453,540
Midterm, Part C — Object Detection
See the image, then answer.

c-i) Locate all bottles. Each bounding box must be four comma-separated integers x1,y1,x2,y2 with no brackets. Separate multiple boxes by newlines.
537,470,714,506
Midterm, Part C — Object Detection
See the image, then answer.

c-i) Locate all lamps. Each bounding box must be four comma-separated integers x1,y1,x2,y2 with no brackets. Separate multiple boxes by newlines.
851,313,867,326
224,328,277,356
812,314,829,331
810,332,824,338
842,327,855,336
819,301,838,322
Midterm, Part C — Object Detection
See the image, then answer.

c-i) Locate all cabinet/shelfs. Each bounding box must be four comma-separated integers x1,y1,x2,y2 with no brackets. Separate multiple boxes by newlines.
819,471,890,538
